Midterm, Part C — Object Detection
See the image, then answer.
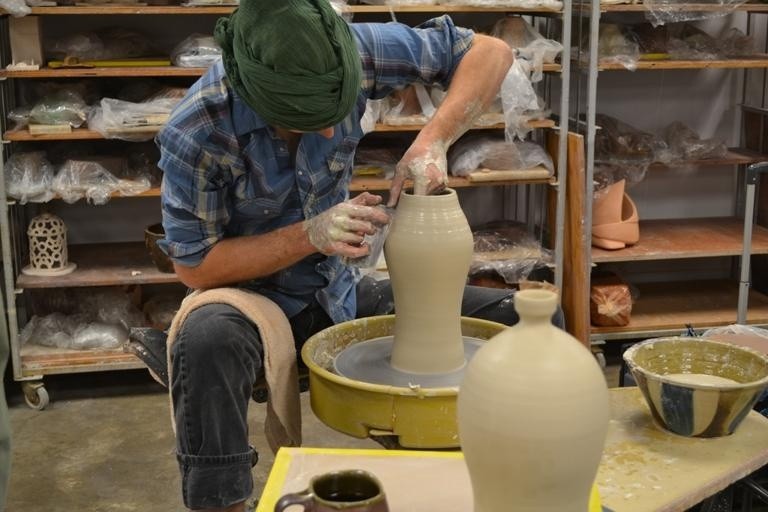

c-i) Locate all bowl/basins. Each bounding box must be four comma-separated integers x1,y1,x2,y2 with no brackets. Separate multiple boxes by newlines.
622,335,768,440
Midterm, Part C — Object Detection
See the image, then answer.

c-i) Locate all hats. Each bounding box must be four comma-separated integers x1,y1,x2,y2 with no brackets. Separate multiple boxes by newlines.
212,0,365,134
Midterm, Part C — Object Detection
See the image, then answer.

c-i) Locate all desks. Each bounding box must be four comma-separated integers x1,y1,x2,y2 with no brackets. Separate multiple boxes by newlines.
585,385,766,512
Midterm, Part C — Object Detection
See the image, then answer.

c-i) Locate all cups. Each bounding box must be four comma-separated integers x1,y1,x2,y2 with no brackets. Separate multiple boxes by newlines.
144,223,176,274
276,467,388,512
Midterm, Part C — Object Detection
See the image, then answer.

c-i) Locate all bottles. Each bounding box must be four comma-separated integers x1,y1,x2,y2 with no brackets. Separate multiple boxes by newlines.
28,214,71,269
378,189,475,375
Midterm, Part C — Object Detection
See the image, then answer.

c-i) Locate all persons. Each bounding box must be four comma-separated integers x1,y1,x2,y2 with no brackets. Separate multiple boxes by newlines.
155,0,515,511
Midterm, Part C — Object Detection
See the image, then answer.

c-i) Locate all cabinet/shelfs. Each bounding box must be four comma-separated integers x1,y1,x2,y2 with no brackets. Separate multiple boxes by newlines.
574,0,766,373
0,0,572,404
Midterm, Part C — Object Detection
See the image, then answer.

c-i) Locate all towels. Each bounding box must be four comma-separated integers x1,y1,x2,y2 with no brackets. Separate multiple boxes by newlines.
163,291,304,462
